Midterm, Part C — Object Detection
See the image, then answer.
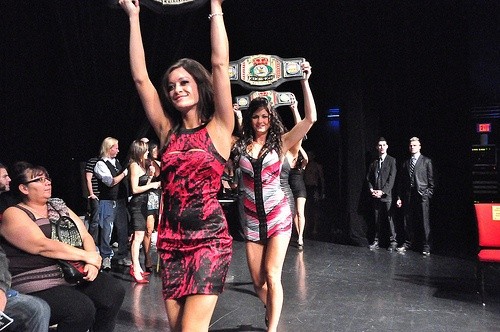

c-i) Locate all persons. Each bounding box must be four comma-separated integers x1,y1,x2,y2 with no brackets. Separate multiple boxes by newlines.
394,137,434,255
119,0,235,332
220,62,318,332
366,137,397,251
0,136,163,332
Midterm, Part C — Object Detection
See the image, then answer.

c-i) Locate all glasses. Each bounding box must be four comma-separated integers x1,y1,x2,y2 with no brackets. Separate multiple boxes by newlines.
22,176,52,185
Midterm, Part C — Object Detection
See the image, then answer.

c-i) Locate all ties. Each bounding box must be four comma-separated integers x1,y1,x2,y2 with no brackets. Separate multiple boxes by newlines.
409,158,416,176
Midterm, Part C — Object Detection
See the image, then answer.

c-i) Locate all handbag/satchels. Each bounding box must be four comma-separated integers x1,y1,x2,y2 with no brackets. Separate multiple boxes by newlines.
56,216,103,285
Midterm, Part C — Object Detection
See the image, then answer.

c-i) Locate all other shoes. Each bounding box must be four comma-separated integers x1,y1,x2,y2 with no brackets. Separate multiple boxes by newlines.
102,257,112,270
367,241,381,248
397,245,411,251
422,250,431,255
297,236,304,246
387,243,398,251
118,256,132,266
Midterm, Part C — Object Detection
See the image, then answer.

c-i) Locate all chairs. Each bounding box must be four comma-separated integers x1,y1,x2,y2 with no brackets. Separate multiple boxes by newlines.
474,203,500,305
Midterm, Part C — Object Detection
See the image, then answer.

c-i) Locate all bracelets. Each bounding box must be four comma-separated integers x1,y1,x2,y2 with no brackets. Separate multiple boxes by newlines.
207,12,224,19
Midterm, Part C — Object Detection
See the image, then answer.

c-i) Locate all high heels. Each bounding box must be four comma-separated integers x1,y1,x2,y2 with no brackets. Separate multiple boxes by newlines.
129,268,150,283
130,265,151,276
144,259,153,272
